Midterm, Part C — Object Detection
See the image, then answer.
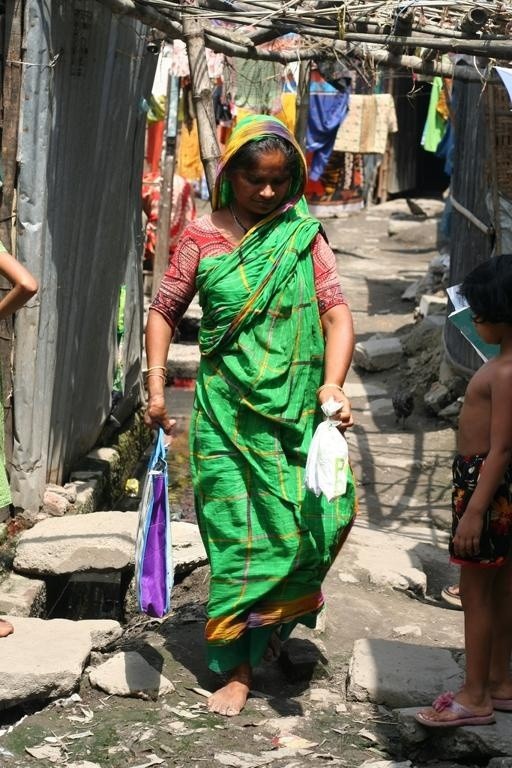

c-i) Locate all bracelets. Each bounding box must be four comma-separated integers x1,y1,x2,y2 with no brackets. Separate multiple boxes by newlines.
145,373,168,386
315,382,346,396
146,366,169,377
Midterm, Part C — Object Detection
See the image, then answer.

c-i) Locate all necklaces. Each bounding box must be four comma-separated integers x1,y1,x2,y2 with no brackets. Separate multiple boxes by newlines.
228,202,248,237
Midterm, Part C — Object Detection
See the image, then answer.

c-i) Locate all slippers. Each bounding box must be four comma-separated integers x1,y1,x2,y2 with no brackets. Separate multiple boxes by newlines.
415,691,495,728
440,583,463,607
459,680,512,712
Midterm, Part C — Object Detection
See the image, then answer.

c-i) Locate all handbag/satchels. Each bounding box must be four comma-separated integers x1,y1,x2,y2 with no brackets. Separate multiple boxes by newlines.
135,467,173,620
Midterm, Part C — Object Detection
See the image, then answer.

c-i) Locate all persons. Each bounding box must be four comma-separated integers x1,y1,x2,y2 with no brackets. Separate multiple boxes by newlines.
414,253,510,734
1,239,42,642
175,76,210,201
143,113,354,719
